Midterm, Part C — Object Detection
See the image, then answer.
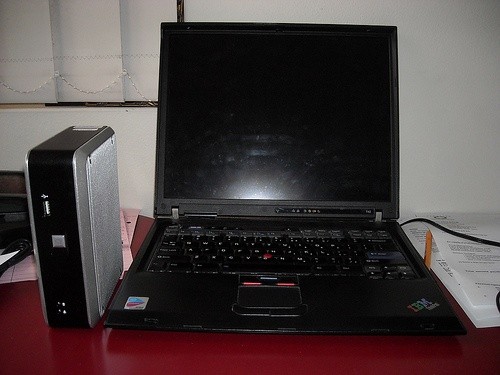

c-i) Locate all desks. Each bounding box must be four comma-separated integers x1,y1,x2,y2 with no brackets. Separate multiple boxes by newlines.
0,204,500,375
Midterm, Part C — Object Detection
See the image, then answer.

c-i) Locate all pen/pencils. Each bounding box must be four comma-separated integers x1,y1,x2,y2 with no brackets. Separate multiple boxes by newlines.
424,228,433,269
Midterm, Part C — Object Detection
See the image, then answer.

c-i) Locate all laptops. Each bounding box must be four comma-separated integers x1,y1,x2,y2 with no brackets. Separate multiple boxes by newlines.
103,22,469,339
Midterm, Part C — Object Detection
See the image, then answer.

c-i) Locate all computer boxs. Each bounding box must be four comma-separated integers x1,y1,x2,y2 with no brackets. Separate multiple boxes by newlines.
25,125,124,330
